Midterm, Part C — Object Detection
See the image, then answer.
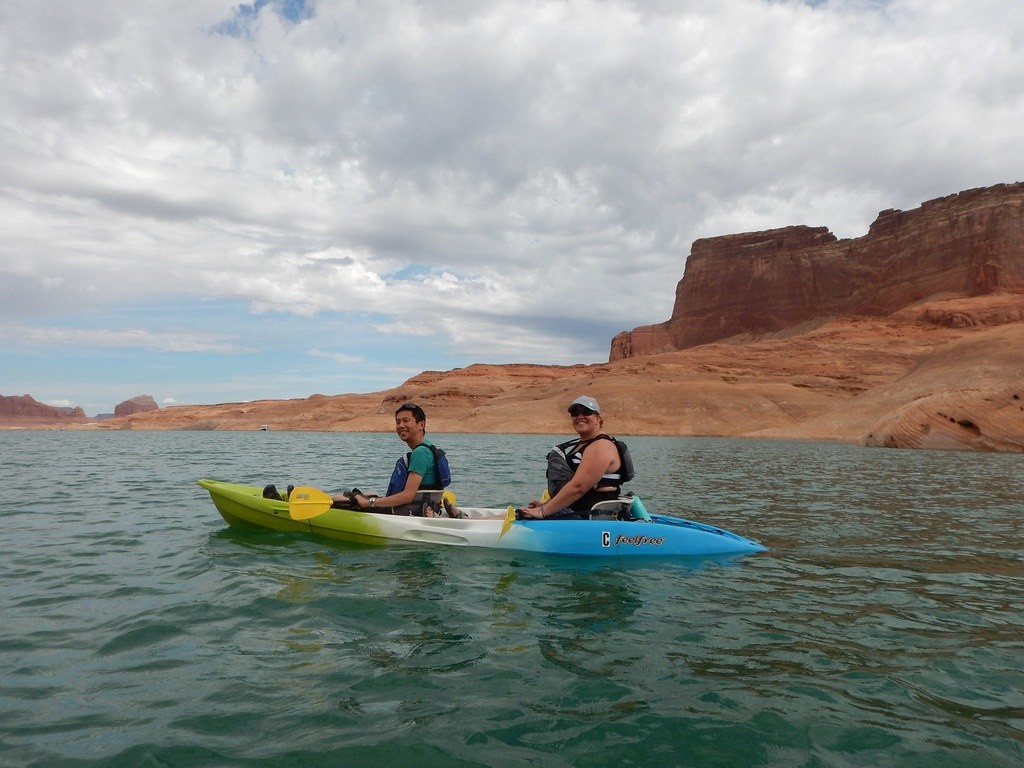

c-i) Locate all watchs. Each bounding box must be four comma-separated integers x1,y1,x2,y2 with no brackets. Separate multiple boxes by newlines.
370,497,375,507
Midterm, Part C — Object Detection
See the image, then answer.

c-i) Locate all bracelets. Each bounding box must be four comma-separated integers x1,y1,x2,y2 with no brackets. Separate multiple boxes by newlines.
539,506,548,518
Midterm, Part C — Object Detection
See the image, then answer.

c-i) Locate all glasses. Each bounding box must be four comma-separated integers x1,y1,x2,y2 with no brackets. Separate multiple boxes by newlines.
570,408,594,417
395,402,423,416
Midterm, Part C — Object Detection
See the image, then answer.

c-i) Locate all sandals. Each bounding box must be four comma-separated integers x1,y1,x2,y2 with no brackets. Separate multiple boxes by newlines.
443,498,469,519
422,503,441,518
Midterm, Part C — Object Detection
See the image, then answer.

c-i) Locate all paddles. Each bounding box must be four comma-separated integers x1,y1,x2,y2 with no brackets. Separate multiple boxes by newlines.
286,485,457,522
495,488,551,544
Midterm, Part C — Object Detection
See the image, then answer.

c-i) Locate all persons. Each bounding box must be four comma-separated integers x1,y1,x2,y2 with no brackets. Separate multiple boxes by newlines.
261,404,444,515
421,396,624,520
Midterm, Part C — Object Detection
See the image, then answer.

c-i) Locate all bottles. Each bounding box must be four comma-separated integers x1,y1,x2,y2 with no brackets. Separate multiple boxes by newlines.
626,490,656,525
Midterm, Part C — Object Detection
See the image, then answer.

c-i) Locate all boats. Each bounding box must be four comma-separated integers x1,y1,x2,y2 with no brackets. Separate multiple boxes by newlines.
198,479,771,569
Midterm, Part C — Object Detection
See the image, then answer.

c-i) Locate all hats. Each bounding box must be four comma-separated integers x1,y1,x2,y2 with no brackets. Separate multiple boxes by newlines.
567,396,600,414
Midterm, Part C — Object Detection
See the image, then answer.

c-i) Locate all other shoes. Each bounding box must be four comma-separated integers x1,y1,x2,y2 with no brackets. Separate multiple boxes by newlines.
287,485,295,498
262,484,282,501
